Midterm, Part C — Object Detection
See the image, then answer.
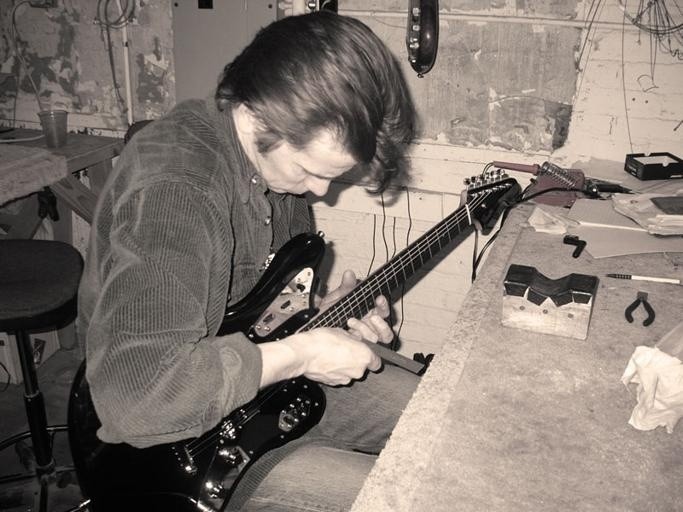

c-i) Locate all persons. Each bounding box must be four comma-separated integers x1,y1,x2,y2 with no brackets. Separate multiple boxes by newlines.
76,9,423,512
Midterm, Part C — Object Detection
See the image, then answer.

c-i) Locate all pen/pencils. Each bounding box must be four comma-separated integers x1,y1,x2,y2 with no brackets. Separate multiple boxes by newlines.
605,274,680,284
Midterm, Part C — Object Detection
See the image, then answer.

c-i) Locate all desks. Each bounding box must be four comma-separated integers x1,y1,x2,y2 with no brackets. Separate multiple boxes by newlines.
341,160,683,512
2,122,131,356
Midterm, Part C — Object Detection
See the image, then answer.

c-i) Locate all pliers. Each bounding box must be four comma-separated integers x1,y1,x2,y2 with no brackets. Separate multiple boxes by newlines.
625,292,655,326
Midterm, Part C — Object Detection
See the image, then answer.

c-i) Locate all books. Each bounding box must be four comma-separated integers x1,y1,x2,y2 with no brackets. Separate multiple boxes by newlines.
567,199,648,233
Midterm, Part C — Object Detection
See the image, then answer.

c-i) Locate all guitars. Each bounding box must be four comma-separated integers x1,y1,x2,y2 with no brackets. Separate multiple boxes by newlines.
67,170,522,512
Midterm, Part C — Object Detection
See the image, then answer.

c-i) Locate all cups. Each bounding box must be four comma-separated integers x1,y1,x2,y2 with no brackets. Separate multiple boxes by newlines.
38,110,68,147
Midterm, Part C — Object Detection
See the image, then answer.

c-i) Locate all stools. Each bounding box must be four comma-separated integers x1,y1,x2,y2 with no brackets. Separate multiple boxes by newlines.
1,238,98,510
